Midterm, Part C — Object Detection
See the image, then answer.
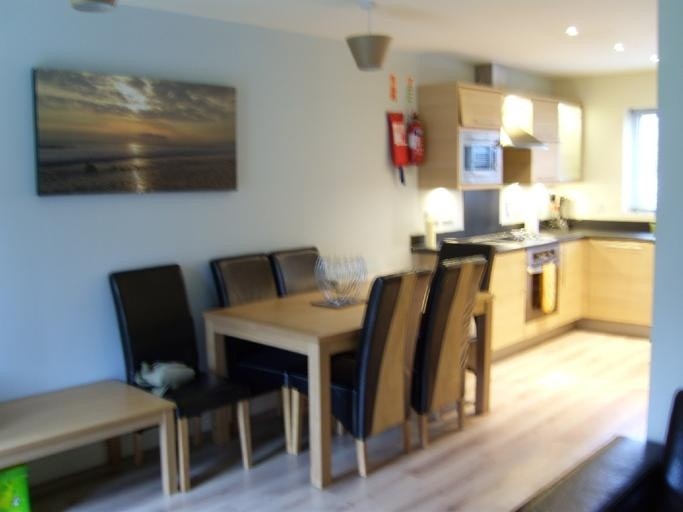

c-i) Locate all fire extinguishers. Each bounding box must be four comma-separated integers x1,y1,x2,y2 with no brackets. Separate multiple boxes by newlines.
407,112,425,166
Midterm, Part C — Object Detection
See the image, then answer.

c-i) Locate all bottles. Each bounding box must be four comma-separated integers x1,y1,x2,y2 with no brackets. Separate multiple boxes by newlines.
424,212,436,250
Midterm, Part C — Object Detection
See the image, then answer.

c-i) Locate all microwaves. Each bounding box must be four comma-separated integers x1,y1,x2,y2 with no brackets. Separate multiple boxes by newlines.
460,127,503,185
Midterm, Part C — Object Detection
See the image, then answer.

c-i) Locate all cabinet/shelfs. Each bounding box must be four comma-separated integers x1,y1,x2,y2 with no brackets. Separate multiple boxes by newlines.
468,253,527,371
584,240,654,331
503,95,584,186
419,83,503,196
500,92,532,146
560,240,583,334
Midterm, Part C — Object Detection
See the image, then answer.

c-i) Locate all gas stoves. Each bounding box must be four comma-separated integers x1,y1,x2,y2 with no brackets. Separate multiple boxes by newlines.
494,230,559,246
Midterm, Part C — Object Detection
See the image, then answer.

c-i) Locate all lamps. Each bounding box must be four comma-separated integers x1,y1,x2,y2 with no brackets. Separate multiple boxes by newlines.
343,1,391,72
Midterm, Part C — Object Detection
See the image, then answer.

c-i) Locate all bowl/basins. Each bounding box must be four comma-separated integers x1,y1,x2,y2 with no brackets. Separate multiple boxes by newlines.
312,254,368,306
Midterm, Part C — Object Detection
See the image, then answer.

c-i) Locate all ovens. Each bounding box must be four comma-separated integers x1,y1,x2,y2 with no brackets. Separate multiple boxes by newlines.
526,243,557,321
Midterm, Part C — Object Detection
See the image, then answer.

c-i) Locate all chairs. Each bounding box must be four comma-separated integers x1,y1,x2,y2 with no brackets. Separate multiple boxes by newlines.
271,244,350,369
402,255,488,453
286,269,434,479
428,240,492,292
108,262,254,492
204,252,338,458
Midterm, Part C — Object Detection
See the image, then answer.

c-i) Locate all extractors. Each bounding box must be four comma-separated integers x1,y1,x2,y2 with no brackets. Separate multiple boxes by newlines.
501,126,548,149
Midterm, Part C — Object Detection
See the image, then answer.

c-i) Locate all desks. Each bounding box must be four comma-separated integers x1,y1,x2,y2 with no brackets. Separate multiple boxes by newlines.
199,275,495,493
509,435,683,510
0,375,175,506
